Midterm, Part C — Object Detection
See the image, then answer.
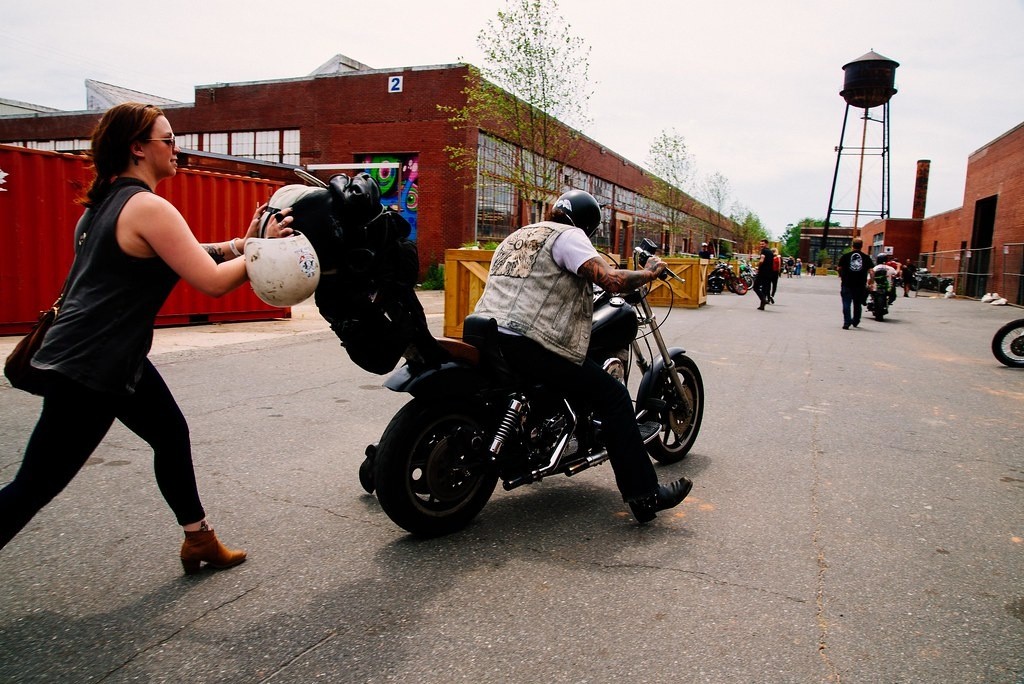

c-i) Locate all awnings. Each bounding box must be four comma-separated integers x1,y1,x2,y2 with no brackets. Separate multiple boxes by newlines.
713,238,737,244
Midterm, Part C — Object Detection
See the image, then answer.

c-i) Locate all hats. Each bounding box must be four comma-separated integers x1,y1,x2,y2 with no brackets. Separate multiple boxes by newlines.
701,242,707,247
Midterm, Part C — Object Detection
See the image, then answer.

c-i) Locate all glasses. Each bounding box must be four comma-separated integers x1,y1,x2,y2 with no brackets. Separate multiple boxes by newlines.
141,133,176,146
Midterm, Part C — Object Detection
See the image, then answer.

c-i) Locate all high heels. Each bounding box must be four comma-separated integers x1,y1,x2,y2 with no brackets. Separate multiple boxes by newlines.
181,528,247,575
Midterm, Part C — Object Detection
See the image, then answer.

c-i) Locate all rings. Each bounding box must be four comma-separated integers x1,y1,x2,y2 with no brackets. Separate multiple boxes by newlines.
277,223,284,230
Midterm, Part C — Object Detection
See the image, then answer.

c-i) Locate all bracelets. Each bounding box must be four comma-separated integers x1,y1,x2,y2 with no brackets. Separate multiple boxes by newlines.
230,237,241,257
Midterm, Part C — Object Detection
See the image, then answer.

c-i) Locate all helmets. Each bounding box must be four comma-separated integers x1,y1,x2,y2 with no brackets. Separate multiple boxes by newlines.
243,230,321,308
876,253,888,264
552,190,602,239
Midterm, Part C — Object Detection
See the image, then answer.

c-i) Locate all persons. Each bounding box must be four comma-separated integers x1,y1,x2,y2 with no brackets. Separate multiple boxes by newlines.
698,243,710,259
865,252,916,308
753,239,773,310
766,247,781,303
0,103,293,573
837,237,874,329
473,189,692,525
778,255,816,278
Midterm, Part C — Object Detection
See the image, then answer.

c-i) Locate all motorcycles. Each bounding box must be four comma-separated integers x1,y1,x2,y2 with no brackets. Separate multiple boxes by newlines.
263,167,705,538
867,269,891,322
705,260,759,295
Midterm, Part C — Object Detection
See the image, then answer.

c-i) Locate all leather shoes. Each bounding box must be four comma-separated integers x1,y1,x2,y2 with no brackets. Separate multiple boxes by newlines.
629,476,694,523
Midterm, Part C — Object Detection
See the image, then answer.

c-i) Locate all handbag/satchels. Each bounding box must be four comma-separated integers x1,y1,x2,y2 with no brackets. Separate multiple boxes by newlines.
4,306,58,397
